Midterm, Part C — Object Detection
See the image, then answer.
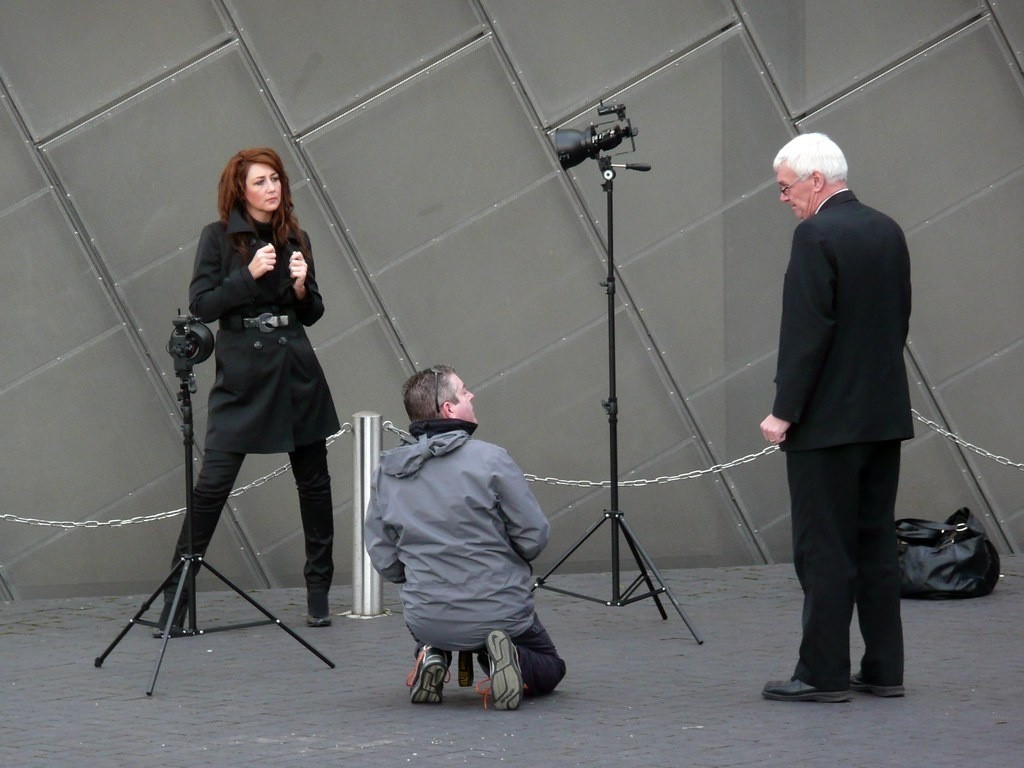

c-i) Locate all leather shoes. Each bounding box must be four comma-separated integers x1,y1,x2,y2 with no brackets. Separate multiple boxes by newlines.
762,677,854,703
849,668,904,697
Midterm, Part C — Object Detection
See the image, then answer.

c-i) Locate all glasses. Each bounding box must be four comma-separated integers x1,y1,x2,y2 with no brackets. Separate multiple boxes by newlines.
780,174,809,194
429,366,442,414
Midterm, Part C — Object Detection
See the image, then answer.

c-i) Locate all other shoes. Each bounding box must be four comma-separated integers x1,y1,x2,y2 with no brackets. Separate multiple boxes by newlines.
406,643,448,706
475,630,528,711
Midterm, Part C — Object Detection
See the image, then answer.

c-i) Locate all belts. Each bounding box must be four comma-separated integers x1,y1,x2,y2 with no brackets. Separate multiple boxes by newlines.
219,315,300,331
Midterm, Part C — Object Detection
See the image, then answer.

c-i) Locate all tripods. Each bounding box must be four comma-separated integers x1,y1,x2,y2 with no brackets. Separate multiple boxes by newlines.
94,372,335,694
527,157,704,646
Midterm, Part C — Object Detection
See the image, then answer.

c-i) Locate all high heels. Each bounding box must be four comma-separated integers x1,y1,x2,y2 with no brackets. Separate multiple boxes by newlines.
306,581,332,627
153,598,194,636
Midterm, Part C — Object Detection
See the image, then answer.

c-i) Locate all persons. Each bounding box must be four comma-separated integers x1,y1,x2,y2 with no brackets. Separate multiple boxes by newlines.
761,130,915,702
364,365,566,710
153,148,342,637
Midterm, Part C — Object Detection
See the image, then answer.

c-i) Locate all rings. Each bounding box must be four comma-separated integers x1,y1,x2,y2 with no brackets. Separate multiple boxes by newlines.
770,440,775,442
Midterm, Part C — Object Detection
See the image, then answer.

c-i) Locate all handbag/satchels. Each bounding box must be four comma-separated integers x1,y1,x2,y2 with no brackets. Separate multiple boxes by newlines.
895,507,1001,600
